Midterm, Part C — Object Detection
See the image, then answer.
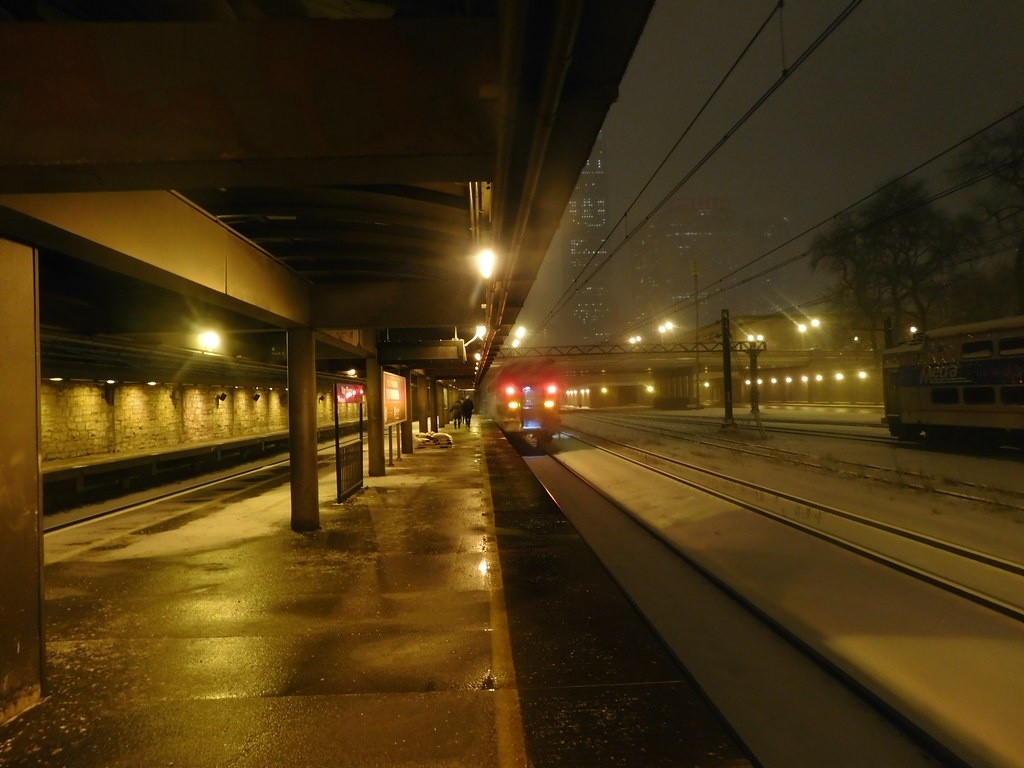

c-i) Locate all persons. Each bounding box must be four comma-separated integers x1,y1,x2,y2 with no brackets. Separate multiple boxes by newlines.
449,395,474,430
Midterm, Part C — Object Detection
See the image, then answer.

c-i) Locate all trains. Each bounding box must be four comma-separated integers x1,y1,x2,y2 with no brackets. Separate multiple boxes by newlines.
484,360,560,453
881,315,1024,441
